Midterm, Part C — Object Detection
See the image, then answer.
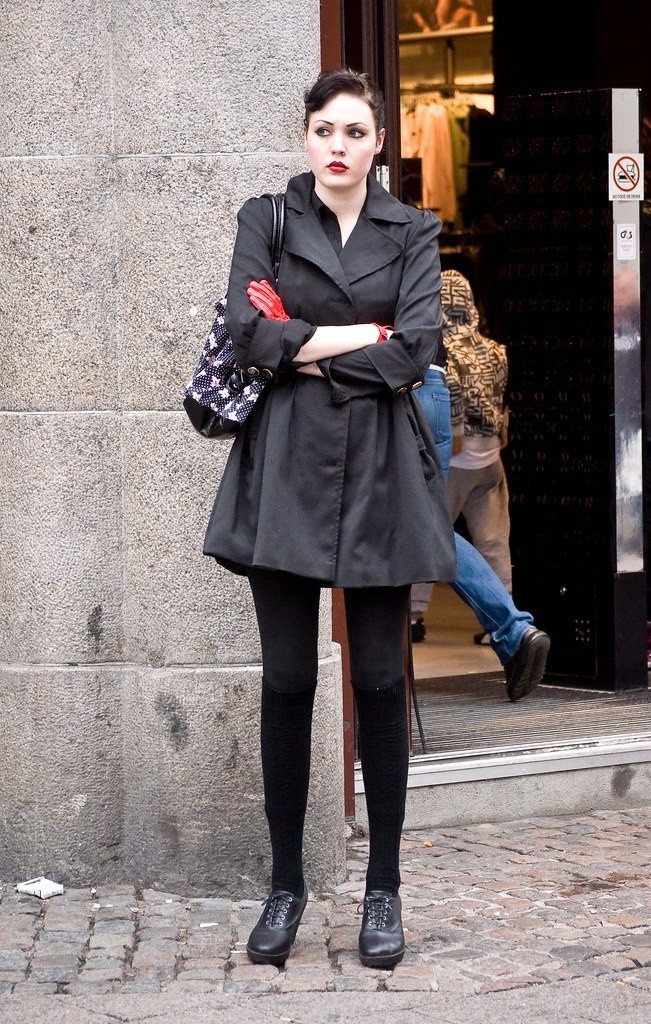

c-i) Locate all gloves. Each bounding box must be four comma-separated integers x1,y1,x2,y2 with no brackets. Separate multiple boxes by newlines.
244,279,290,324
375,323,396,345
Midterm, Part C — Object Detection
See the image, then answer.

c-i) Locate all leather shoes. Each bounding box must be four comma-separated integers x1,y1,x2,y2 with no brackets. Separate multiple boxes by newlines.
246,880,308,964
356,890,404,968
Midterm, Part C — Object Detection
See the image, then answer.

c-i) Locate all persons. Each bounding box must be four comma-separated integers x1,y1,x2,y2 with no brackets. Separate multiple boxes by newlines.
410,341,552,703
203,67,458,968
411,271,511,644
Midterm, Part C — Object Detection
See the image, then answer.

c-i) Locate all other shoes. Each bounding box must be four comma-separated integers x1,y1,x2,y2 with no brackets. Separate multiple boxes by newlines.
473,631,494,645
503,627,550,702
412,618,426,643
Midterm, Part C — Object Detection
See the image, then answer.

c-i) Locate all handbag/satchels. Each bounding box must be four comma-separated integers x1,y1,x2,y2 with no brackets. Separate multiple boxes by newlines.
183,193,287,439
